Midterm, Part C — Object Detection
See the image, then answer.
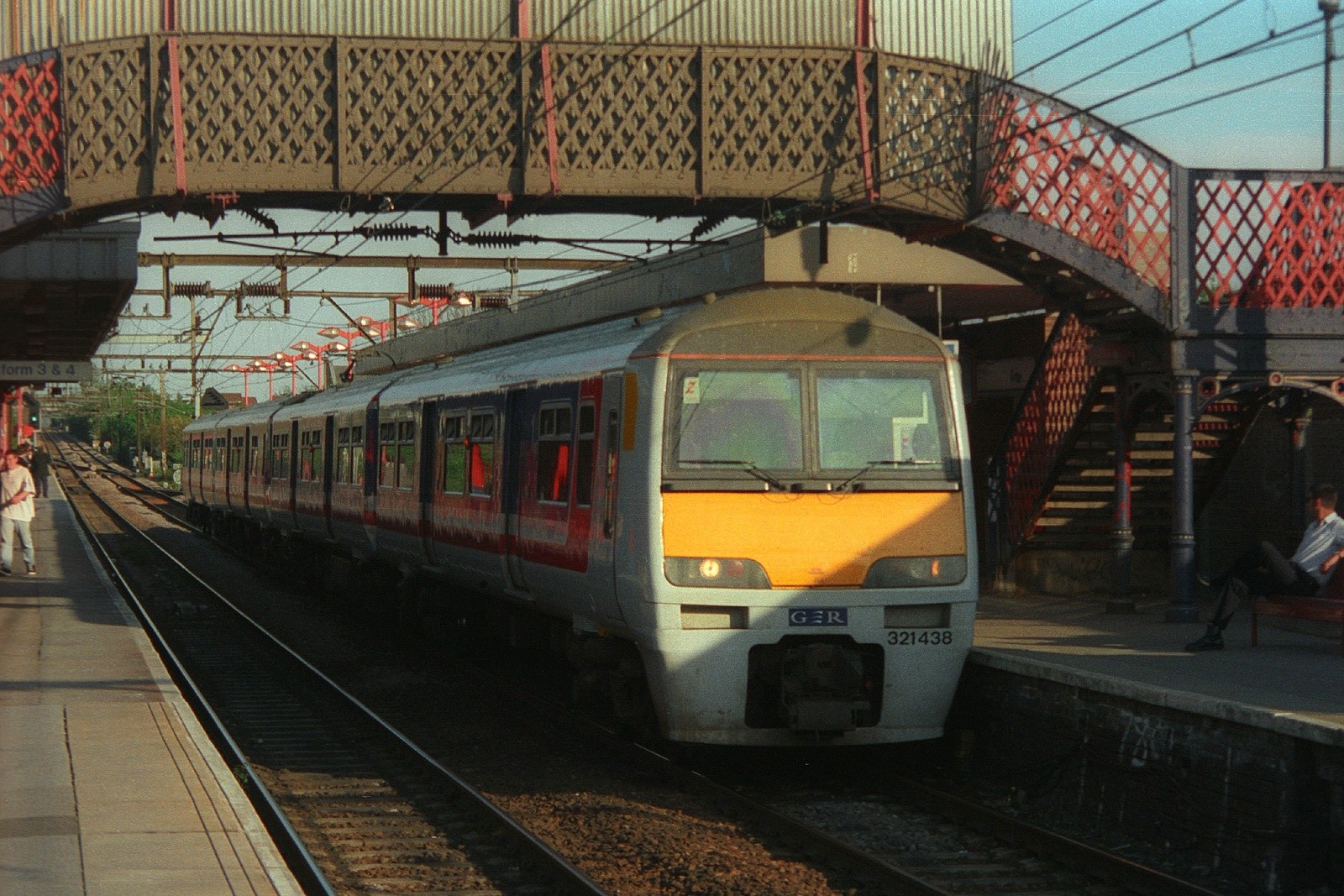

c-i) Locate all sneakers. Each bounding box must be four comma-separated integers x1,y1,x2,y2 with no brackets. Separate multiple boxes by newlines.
0,566,13,576
27,564,37,575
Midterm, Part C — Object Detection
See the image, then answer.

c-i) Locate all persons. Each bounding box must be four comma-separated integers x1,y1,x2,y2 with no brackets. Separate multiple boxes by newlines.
16,443,29,469
0,448,39,576
1183,483,1344,652
33,445,52,499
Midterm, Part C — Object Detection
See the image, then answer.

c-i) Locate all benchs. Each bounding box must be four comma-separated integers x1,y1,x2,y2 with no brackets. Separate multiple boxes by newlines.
1249,544,1344,648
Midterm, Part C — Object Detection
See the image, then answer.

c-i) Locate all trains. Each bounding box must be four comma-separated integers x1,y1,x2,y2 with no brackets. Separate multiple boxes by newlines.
184,284,978,766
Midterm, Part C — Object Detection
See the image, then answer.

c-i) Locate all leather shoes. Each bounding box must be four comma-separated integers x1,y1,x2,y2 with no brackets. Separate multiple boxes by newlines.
1197,571,1224,587
1184,636,1226,652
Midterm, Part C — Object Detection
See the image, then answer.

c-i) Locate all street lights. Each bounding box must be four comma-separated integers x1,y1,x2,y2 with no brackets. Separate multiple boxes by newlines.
224,292,481,407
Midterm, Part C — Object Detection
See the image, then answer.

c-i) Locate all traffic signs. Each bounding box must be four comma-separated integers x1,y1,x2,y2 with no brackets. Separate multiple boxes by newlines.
0,359,93,382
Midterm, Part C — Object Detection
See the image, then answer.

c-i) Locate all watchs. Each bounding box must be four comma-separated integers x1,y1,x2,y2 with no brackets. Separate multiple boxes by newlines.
1335,550,1343,558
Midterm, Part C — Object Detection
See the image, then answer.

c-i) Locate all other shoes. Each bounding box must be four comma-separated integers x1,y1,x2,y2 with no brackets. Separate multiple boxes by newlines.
44,494,48,498
33,494,40,497
